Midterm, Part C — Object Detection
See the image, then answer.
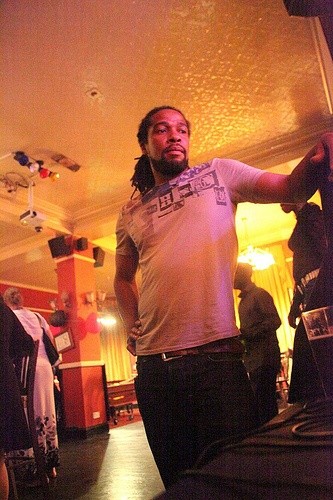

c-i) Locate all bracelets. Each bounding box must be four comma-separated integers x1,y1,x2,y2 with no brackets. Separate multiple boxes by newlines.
54,380,59,383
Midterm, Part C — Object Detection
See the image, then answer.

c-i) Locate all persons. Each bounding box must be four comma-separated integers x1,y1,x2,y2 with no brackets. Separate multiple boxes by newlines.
281,200,321,329
52,366,60,417
3,288,59,482
113,106,333,485
233,262,282,420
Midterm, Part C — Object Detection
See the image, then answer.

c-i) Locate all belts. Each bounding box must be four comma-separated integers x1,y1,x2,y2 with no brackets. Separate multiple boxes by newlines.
155,340,246,361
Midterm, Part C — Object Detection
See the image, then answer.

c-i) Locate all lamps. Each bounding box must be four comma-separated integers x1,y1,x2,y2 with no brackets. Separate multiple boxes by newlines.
13,151,60,182
86,290,107,313
238,217,275,269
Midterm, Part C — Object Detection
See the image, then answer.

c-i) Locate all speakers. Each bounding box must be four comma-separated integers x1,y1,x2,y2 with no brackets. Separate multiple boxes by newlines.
93,247,105,267
48,235,73,258
78,237,88,250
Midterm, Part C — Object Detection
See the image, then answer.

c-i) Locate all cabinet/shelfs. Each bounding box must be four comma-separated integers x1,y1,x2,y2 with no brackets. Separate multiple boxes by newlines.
108,383,137,424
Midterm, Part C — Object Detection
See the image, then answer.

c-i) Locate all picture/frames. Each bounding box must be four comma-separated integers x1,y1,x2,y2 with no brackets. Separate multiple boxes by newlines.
53,328,74,354
